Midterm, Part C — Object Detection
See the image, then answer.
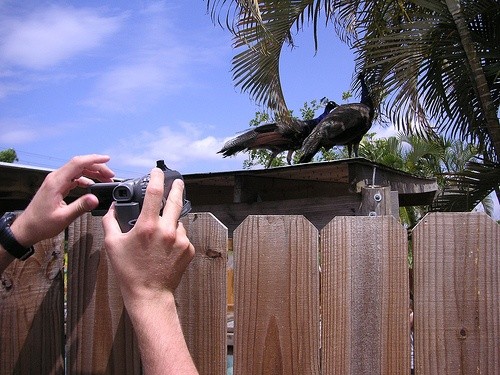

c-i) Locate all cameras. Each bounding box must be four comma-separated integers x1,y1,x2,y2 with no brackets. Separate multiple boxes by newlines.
86,160,192,233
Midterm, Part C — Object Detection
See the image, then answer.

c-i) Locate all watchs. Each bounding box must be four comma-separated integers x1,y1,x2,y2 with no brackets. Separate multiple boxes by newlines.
0,211,35,262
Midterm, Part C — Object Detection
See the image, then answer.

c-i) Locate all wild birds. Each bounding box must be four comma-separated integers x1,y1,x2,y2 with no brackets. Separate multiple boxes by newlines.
216,72,374,169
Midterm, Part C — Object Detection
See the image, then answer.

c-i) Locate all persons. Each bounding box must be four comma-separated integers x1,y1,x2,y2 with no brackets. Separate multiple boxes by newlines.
408,298,415,369
1,153,199,375
